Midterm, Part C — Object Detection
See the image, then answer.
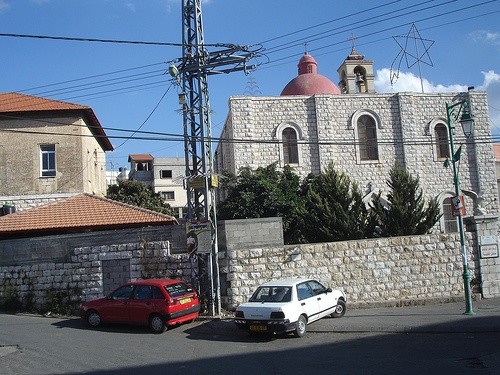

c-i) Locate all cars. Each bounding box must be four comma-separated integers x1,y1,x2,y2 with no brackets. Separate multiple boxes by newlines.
233,277,347,337
82,278,200,332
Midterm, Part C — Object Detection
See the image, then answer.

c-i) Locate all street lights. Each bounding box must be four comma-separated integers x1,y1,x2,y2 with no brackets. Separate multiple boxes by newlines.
443,97,475,316
